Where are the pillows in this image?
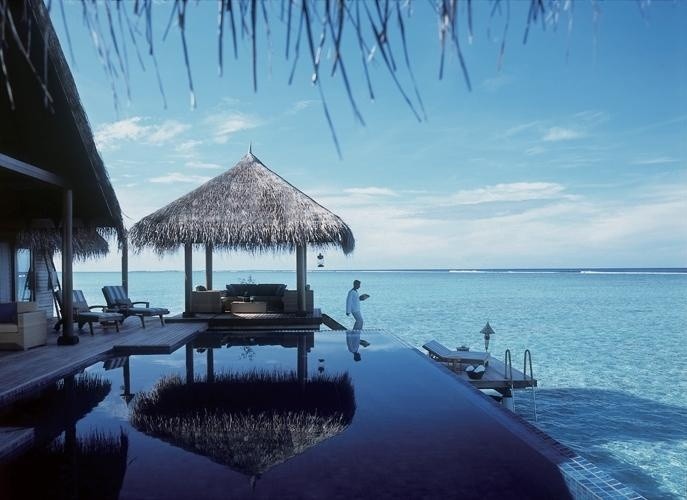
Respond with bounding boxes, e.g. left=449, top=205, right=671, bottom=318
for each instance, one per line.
left=0, top=302, right=18, bottom=324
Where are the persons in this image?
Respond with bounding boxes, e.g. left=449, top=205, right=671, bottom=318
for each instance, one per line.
left=346, top=280, right=368, bottom=330
left=346, top=330, right=371, bottom=361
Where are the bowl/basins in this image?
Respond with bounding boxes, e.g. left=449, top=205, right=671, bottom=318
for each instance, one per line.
left=466, top=371, right=484, bottom=380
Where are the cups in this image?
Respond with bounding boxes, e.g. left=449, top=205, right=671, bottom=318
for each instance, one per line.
left=250, top=296, right=253, bottom=303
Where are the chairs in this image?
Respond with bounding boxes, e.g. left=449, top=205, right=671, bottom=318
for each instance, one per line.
left=0, top=300, right=49, bottom=351
left=54, top=285, right=171, bottom=337
left=422, top=339, right=491, bottom=374
left=191, top=285, right=223, bottom=314
left=282, top=285, right=314, bottom=314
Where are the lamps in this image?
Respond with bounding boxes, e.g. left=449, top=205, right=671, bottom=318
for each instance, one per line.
left=479, top=321, right=496, bottom=352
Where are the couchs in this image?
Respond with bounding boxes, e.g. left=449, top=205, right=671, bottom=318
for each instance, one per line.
left=220, top=283, right=287, bottom=310
